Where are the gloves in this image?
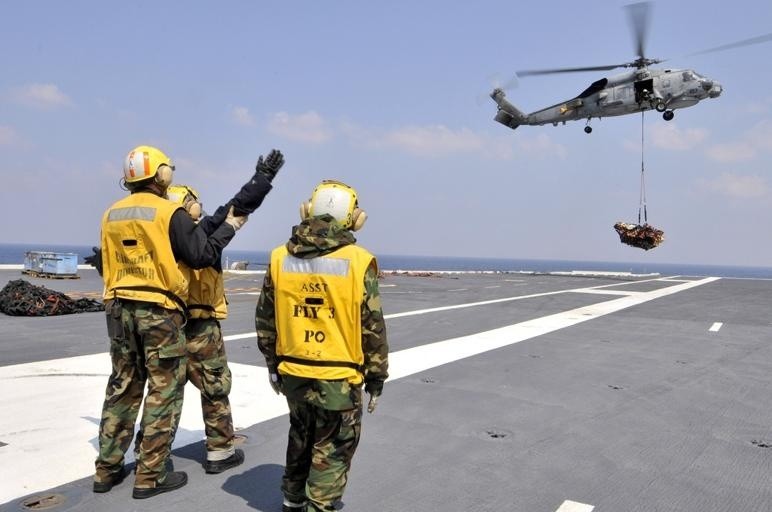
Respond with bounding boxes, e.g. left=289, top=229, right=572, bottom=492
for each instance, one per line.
left=225, top=204, right=250, bottom=233
left=83, top=245, right=101, bottom=269
left=365, top=387, right=381, bottom=413
left=267, top=372, right=287, bottom=396
left=256, top=147, right=286, bottom=184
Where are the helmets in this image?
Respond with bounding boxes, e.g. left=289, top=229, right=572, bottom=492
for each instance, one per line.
left=309, top=178, right=359, bottom=230
left=166, top=182, right=201, bottom=208
left=123, top=145, right=176, bottom=184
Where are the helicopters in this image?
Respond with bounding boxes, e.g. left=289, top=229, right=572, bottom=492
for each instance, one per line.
left=490, top=0, right=772, bottom=133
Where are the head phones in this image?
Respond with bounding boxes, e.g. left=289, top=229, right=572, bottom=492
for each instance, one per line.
left=300, top=199, right=366, bottom=232
left=123, top=165, right=173, bottom=190
left=186, top=200, right=202, bottom=221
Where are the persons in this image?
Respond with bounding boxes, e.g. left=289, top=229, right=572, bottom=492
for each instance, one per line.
left=83, top=145, right=285, bottom=473
left=93, top=144, right=247, bottom=499
left=256, top=180, right=388, bottom=511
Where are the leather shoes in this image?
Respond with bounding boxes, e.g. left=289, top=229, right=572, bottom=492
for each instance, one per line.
left=206, top=447, right=246, bottom=474
left=130, top=469, right=189, bottom=499
left=93, top=466, right=125, bottom=493
